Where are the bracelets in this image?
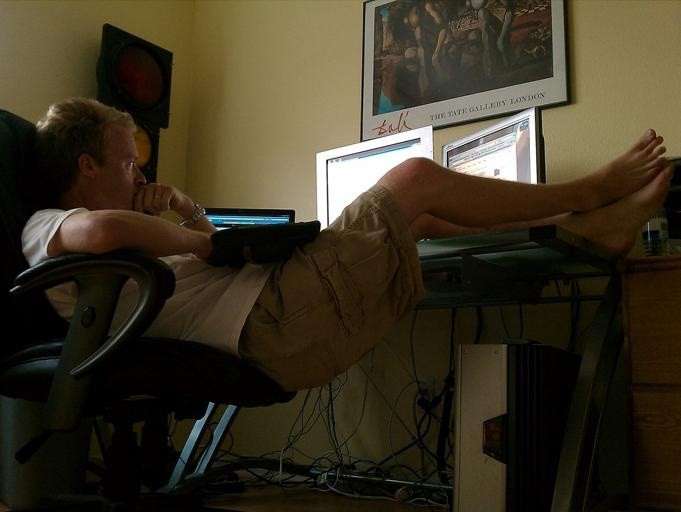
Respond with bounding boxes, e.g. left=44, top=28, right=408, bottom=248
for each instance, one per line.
left=180, top=203, right=206, bottom=227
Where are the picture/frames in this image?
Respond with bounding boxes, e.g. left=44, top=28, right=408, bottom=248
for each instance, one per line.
left=361, top=1, right=572, bottom=143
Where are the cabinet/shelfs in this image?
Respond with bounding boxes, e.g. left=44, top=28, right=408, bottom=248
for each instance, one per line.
left=606, top=246, right=679, bottom=510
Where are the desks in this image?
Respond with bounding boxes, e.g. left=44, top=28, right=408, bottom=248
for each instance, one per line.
left=161, top=221, right=630, bottom=509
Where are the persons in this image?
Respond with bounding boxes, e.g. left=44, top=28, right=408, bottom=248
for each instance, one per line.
left=16, top=92, right=679, bottom=393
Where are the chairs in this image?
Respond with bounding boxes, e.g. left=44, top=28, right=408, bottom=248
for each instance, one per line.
left=0, top=107, right=295, bottom=508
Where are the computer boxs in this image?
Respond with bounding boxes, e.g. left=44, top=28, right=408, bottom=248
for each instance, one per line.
left=452, top=340, right=580, bottom=512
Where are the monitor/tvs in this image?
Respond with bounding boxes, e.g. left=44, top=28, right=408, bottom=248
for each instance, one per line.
left=99, top=22, right=174, bottom=130
left=314, top=124, right=436, bottom=235
left=442, top=105, right=548, bottom=192
left=197, top=206, right=298, bottom=238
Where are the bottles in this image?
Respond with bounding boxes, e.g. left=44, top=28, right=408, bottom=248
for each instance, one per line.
left=638, top=212, right=670, bottom=258
